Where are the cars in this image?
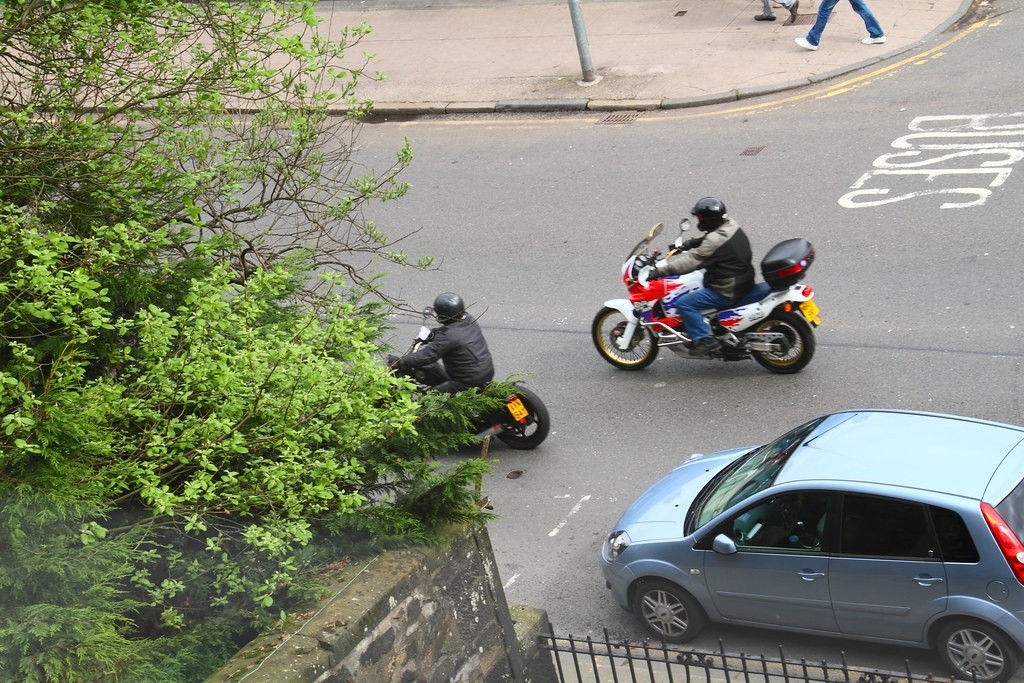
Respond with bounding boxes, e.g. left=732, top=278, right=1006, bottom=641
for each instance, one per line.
left=600, top=409, right=1024, bottom=683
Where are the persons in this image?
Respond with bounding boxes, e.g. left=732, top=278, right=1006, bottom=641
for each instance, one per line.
left=385, top=292, right=495, bottom=405
left=645, top=197, right=755, bottom=357
left=795, top=0, right=886, bottom=50
left=754, top=0, right=799, bottom=23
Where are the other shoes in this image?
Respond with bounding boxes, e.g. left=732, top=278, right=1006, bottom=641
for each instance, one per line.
left=754, top=14, right=776, bottom=21
left=789, top=0, right=799, bottom=23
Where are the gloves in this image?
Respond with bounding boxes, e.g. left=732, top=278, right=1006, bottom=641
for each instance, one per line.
left=646, top=269, right=665, bottom=281
left=387, top=355, right=400, bottom=366
left=668, top=243, right=685, bottom=255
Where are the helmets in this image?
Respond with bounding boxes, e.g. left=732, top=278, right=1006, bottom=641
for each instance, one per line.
left=691, top=197, right=727, bottom=231
left=434, top=293, right=465, bottom=325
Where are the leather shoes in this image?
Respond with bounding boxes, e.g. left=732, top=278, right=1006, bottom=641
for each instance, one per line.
left=688, top=338, right=722, bottom=357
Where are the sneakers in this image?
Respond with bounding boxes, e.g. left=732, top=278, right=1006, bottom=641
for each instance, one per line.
left=795, top=38, right=817, bottom=51
left=862, top=37, right=886, bottom=44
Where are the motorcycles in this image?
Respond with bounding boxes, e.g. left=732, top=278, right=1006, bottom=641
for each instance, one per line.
left=373, top=306, right=551, bottom=450
left=591, top=218, right=824, bottom=374
